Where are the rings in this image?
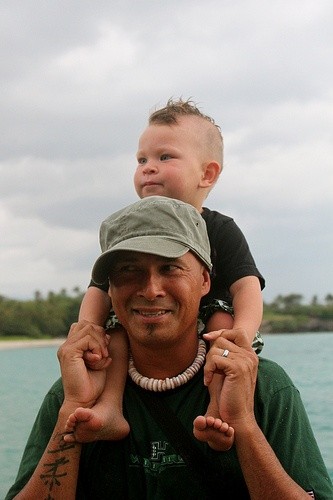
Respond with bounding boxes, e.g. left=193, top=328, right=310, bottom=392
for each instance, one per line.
left=223, top=349, right=230, bottom=358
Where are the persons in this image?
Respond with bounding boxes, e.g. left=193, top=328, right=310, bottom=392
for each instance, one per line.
left=62, top=100, right=266, bottom=453
left=5, top=195, right=333, bottom=500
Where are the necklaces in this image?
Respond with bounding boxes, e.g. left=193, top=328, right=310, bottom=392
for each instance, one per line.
left=126, top=337, right=207, bottom=392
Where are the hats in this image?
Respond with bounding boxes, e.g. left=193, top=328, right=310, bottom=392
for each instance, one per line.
left=91, top=196, right=213, bottom=284
